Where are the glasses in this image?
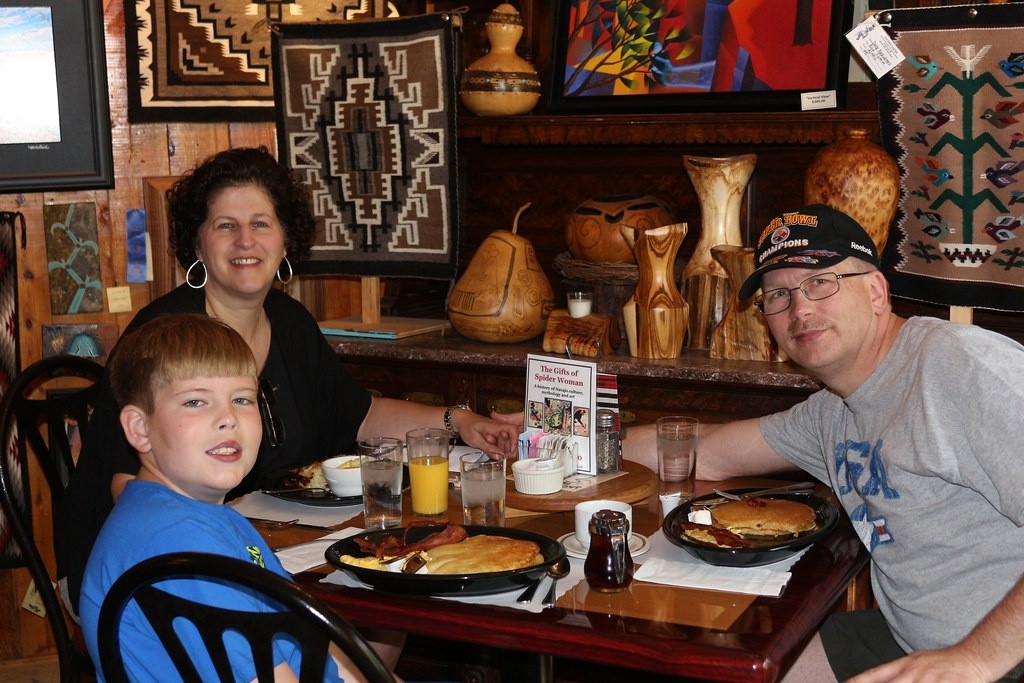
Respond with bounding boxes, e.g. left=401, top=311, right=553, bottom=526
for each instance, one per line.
left=755, top=270, right=874, bottom=316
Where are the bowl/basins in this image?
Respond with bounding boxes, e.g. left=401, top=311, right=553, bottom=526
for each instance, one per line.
left=662, top=488, right=833, bottom=567
left=321, top=455, right=376, bottom=496
left=575, top=499, right=632, bottom=551
left=511, top=458, right=565, bottom=495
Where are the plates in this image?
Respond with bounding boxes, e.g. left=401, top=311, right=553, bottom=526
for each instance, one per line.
left=324, top=523, right=567, bottom=596
left=402, top=445, right=489, bottom=472
left=260, top=465, right=410, bottom=507
left=557, top=532, right=652, bottom=559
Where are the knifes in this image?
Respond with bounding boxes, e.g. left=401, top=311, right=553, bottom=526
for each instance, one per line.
left=693, top=490, right=814, bottom=506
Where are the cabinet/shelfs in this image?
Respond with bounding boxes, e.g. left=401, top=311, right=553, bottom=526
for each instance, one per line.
left=333, top=106, right=884, bottom=399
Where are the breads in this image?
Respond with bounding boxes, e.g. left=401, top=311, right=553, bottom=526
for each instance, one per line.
left=295, top=461, right=327, bottom=492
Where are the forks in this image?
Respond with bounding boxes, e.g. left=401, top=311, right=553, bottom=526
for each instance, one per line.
left=714, top=483, right=815, bottom=502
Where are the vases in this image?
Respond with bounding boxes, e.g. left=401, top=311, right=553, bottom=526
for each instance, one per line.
left=622, top=221, right=690, bottom=358
left=680, top=153, right=759, bottom=353
left=804, top=125, right=902, bottom=255
left=709, top=244, right=788, bottom=362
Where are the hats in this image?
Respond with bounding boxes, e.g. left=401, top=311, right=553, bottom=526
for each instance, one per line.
left=737, top=202, right=879, bottom=303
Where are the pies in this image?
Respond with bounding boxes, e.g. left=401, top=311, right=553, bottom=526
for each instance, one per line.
left=417, top=534, right=544, bottom=573
left=689, top=497, right=816, bottom=535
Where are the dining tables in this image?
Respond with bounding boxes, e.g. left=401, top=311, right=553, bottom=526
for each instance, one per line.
left=252, top=472, right=870, bottom=683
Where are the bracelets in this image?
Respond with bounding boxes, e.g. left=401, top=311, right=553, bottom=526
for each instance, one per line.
left=444, top=404, right=473, bottom=437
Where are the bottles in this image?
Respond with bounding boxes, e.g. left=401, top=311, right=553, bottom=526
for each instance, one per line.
left=596, top=408, right=619, bottom=474
left=584, top=511, right=633, bottom=592
left=805, top=123, right=900, bottom=259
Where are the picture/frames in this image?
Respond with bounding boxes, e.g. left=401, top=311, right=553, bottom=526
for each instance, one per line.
left=141, top=174, right=327, bottom=324
left=0, top=0, right=116, bottom=195
left=545, top=0, right=854, bottom=112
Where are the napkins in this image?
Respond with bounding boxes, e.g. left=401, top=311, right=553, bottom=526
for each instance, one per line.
left=272, top=526, right=366, bottom=576
left=631, top=556, right=792, bottom=597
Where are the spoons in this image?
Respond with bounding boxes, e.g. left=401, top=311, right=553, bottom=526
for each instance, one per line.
left=249, top=519, right=301, bottom=531
left=542, top=558, right=570, bottom=607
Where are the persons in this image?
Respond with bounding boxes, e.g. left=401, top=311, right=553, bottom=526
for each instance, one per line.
left=484, top=202, right=1024, bottom=682
left=79, top=313, right=405, bottom=683
left=58, top=144, right=518, bottom=591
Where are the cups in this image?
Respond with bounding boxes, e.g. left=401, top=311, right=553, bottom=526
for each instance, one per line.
left=655, top=417, right=697, bottom=501
left=358, top=437, right=403, bottom=528
left=459, top=452, right=506, bottom=527
left=567, top=291, right=592, bottom=317
left=406, top=427, right=450, bottom=522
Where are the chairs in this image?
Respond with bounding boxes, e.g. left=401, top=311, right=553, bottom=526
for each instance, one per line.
left=0, top=355, right=143, bottom=683
left=95, top=551, right=395, bottom=683
